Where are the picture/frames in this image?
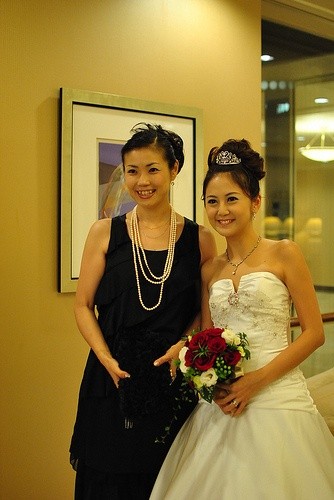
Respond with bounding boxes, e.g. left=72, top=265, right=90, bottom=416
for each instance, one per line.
left=59, top=88, right=204, bottom=294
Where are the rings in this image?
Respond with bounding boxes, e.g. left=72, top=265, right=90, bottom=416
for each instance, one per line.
left=232, top=400, right=239, bottom=408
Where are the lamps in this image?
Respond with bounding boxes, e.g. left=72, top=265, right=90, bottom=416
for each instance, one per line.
left=299, top=134, right=334, bottom=163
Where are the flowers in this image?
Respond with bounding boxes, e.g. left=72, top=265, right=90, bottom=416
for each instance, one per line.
left=177, top=323, right=252, bottom=403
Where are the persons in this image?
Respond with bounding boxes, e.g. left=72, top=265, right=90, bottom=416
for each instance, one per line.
left=68, top=123, right=220, bottom=500
left=148, top=136, right=334, bottom=500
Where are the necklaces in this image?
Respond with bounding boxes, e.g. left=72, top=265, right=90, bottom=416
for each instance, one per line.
left=224, top=234, right=262, bottom=275
left=128, top=201, right=178, bottom=310
